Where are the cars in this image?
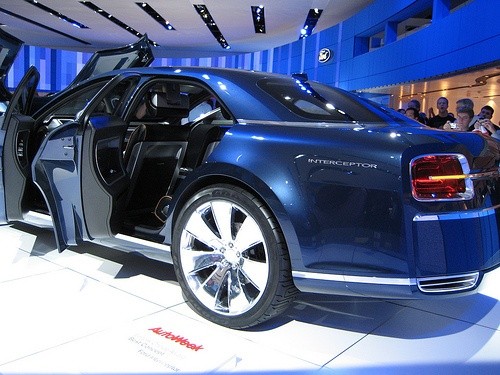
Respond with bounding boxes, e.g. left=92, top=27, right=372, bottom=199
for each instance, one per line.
left=1, top=65, right=500, bottom=331
left=0, top=27, right=155, bottom=146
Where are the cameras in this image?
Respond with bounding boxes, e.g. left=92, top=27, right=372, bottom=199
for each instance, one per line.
left=449, top=123, right=457, bottom=128
left=479, top=119, right=490, bottom=125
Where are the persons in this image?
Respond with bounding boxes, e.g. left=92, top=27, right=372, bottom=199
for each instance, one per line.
left=398, top=97, right=500, bottom=140
